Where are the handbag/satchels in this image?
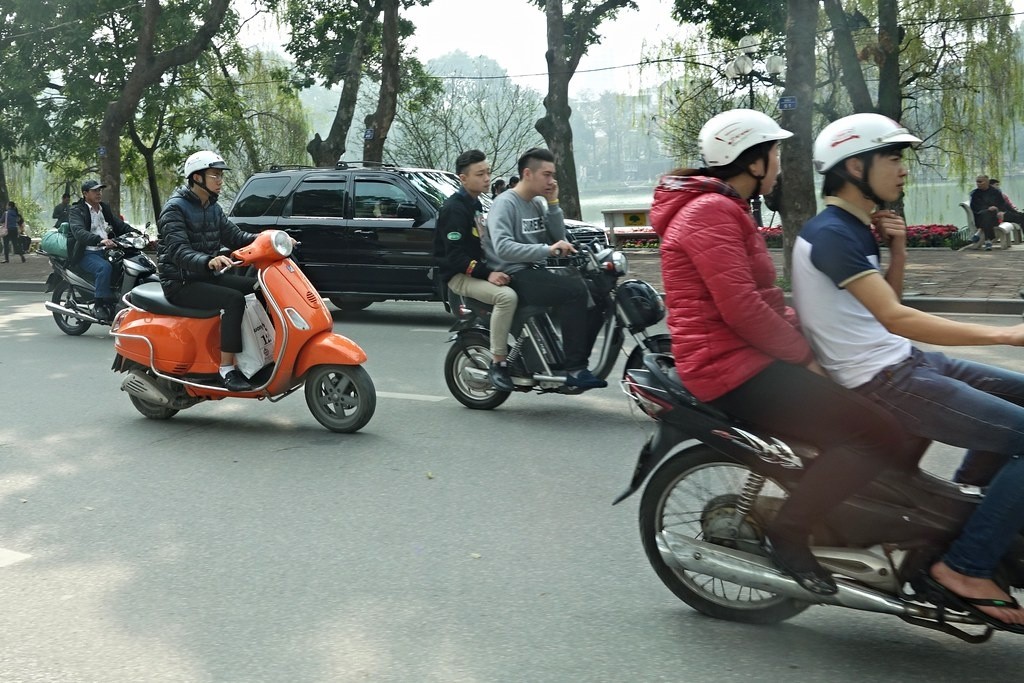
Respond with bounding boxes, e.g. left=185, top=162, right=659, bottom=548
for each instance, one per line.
left=0, top=226, right=8, bottom=237
left=221, top=293, right=276, bottom=379
left=19, top=233, right=31, bottom=250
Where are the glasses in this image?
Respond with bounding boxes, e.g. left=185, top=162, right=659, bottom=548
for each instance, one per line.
left=207, top=173, right=225, bottom=181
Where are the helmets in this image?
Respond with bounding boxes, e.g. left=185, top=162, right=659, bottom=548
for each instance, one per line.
left=813, top=112, right=923, bottom=172
left=185, top=151, right=232, bottom=178
left=697, top=108, right=794, bottom=166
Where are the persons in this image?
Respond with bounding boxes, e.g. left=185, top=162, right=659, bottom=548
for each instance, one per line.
left=433, top=146, right=609, bottom=391
left=649, top=109, right=909, bottom=595
left=157, top=150, right=297, bottom=392
left=792, top=113, right=1024, bottom=633
left=970, top=173, right=1024, bottom=250
left=0, top=180, right=149, bottom=321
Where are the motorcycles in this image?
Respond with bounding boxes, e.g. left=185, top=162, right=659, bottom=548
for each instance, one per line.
left=35, top=221, right=161, bottom=335
left=620, top=290, right=1024, bottom=645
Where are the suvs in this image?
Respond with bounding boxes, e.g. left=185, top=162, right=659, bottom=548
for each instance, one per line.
left=226, top=161, right=609, bottom=314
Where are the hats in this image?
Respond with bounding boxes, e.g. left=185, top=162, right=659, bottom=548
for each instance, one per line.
left=81, top=179, right=107, bottom=190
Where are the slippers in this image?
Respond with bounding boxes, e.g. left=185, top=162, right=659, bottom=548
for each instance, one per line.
left=927, top=562, right=1024, bottom=636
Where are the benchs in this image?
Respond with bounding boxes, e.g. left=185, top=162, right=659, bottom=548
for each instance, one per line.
left=601, top=208, right=662, bottom=247
left=1011, top=222, right=1023, bottom=245
left=25, top=225, right=43, bottom=253
left=959, top=200, right=1014, bottom=250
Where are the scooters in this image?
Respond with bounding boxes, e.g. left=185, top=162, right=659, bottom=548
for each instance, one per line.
left=108, top=228, right=377, bottom=433
left=444, top=239, right=672, bottom=410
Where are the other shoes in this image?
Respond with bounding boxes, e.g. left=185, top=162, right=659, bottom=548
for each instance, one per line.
left=21, top=256, right=25, bottom=262
left=92, top=305, right=109, bottom=319
left=985, top=242, right=992, bottom=250
left=971, top=234, right=980, bottom=243
left=2, top=259, right=10, bottom=263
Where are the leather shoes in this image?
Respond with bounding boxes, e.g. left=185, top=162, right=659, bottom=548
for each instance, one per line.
left=218, top=369, right=250, bottom=391
left=565, top=370, right=608, bottom=389
left=488, top=361, right=516, bottom=392
left=772, top=529, right=839, bottom=596
